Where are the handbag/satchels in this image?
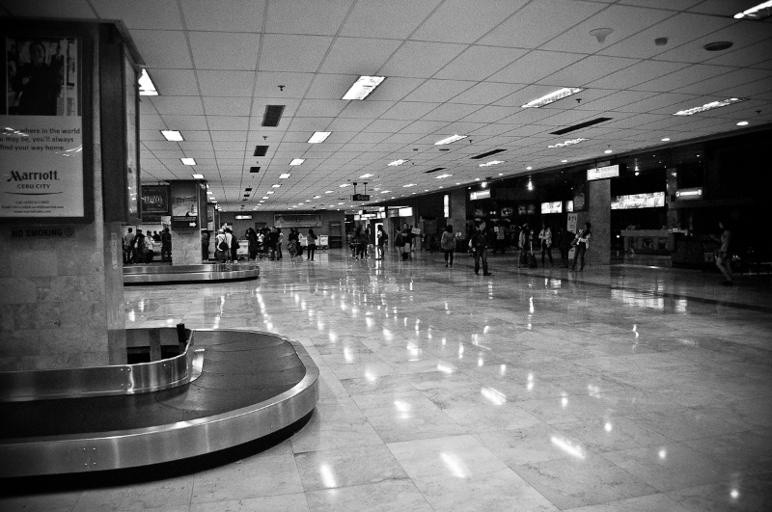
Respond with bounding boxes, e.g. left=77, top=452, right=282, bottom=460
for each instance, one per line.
left=235, top=243, right=240, bottom=250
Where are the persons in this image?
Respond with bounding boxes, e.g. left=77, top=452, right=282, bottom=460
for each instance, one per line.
left=440, top=224, right=457, bottom=268
left=202, top=230, right=210, bottom=262
left=245, top=224, right=304, bottom=261
left=307, top=229, right=317, bottom=260
left=213, top=223, right=240, bottom=264
left=346, top=221, right=422, bottom=260
left=7, top=37, right=68, bottom=116
left=516, top=221, right=592, bottom=271
left=121, top=227, right=172, bottom=264
left=470, top=219, right=492, bottom=278
left=709, top=220, right=734, bottom=287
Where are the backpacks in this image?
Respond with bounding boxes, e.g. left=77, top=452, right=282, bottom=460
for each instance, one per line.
left=218, top=237, right=228, bottom=250
